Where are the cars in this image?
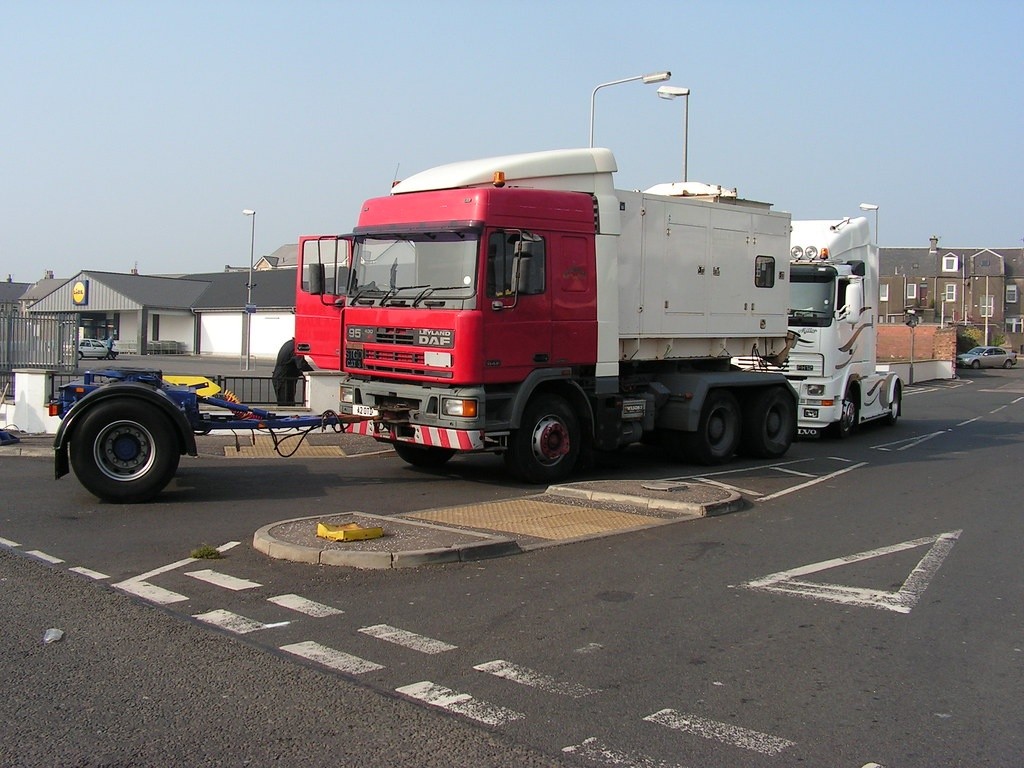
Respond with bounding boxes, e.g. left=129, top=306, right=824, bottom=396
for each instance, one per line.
left=956, top=345, right=1017, bottom=369
left=48, top=338, right=119, bottom=360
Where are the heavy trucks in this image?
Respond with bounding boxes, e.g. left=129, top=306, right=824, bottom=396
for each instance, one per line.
left=294, top=148, right=800, bottom=486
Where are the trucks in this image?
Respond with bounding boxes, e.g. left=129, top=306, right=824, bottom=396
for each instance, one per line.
left=729, top=215, right=904, bottom=440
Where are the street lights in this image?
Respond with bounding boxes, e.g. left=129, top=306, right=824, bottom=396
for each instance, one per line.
left=243, top=209, right=256, bottom=374
left=860, top=202, right=880, bottom=245
left=656, top=85, right=690, bottom=183
left=589, top=71, right=672, bottom=147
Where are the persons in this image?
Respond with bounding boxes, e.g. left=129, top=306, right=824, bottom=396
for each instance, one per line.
left=272, top=338, right=313, bottom=406
left=104, top=335, right=116, bottom=360
